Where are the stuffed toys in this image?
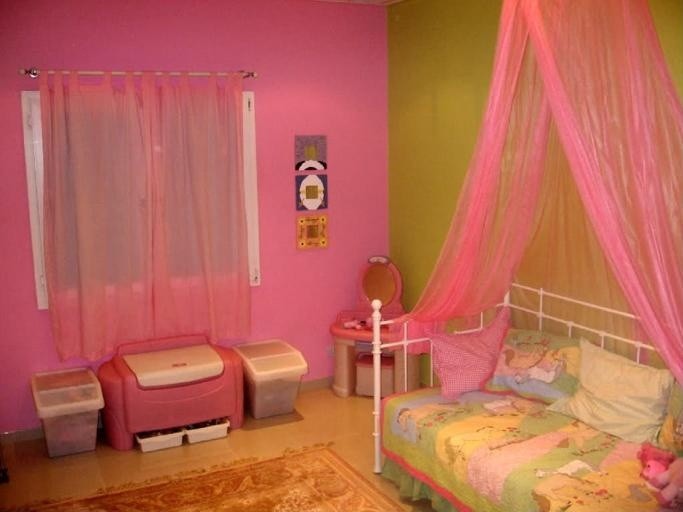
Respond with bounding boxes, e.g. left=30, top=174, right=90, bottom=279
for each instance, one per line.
left=637, top=439, right=683, bottom=509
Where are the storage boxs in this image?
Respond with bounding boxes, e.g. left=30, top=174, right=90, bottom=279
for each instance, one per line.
left=30, top=332, right=309, bottom=458
left=356, top=354, right=393, bottom=398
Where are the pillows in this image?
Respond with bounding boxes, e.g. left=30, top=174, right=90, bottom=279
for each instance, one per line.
left=424, top=305, right=674, bottom=447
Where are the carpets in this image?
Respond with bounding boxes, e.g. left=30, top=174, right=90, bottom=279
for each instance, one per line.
left=11, top=441, right=409, bottom=512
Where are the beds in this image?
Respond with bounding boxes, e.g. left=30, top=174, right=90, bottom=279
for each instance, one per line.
left=372, top=283, right=683, bottom=511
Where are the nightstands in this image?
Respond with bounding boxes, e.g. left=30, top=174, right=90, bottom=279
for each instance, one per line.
left=330, top=318, right=419, bottom=397
